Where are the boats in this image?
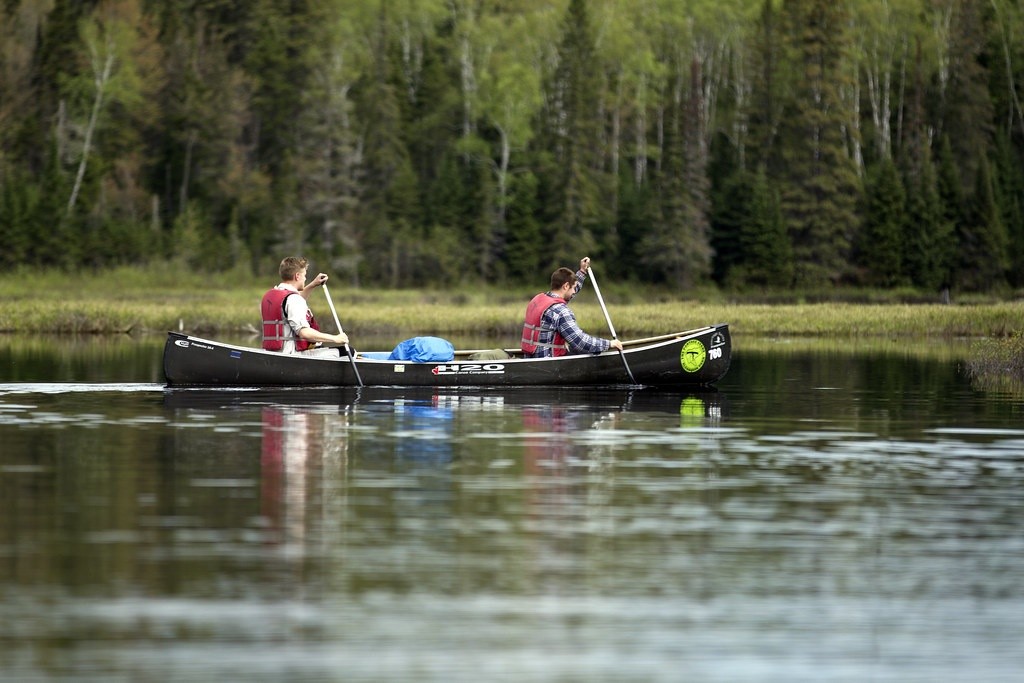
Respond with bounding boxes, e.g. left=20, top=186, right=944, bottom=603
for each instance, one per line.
left=158, top=320, right=735, bottom=389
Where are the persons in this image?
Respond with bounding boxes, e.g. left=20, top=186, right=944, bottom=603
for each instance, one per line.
left=519, top=255, right=623, bottom=358
left=261, top=256, right=359, bottom=360
